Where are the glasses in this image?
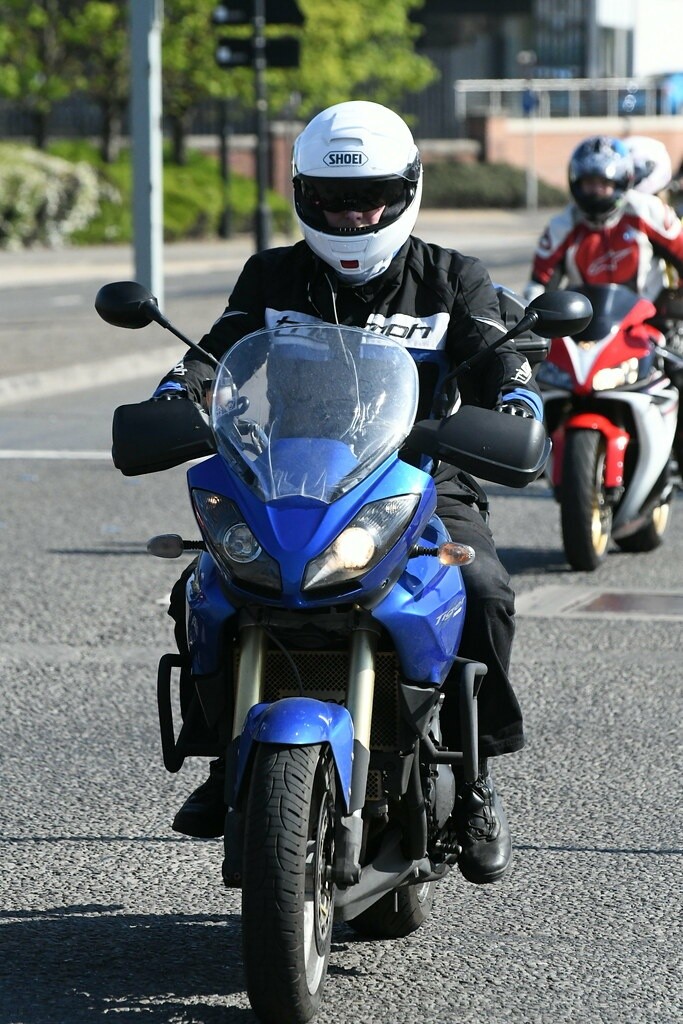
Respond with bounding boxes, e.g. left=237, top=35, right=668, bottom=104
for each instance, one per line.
left=314, top=182, right=393, bottom=214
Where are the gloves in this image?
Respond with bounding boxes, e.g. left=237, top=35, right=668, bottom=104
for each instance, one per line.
left=142, top=394, right=183, bottom=402
left=492, top=405, right=537, bottom=420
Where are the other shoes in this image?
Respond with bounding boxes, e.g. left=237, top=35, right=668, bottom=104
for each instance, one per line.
left=453, top=769, right=513, bottom=885
left=172, top=760, right=224, bottom=838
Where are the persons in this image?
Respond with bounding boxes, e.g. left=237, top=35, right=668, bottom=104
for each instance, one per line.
left=146, top=101, right=547, bottom=887
left=519, top=135, right=683, bottom=493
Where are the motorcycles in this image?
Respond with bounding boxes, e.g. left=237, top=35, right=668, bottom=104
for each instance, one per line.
left=88, top=277, right=598, bottom=1022
left=487, top=249, right=682, bottom=573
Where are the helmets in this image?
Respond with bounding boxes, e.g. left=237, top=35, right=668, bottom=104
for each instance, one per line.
left=618, top=136, right=671, bottom=196
left=290, top=101, right=422, bottom=277
left=567, top=135, right=635, bottom=219
